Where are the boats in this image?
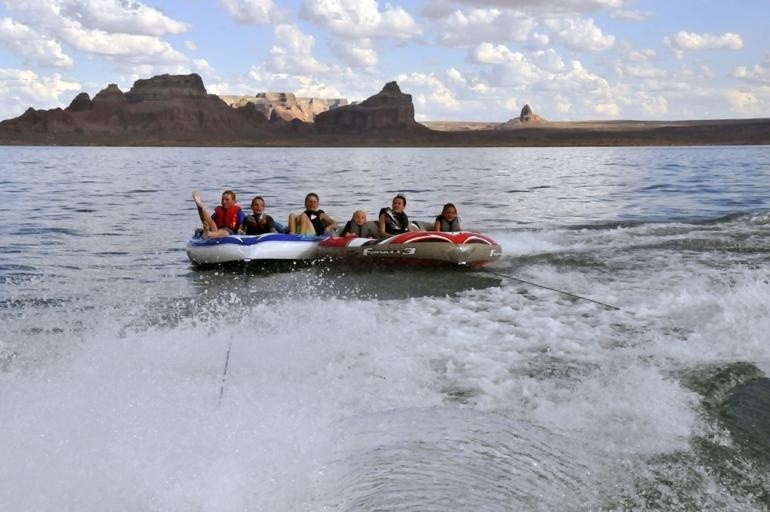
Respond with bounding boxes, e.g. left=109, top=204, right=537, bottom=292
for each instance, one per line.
left=318, top=221, right=501, bottom=267
left=185, top=221, right=329, bottom=266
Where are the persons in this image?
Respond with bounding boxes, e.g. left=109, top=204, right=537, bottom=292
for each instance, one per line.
left=379, top=194, right=409, bottom=239
left=434, top=203, right=460, bottom=232
left=240, top=197, right=280, bottom=235
left=341, top=210, right=377, bottom=240
left=192, top=190, right=246, bottom=237
left=288, top=193, right=337, bottom=237
